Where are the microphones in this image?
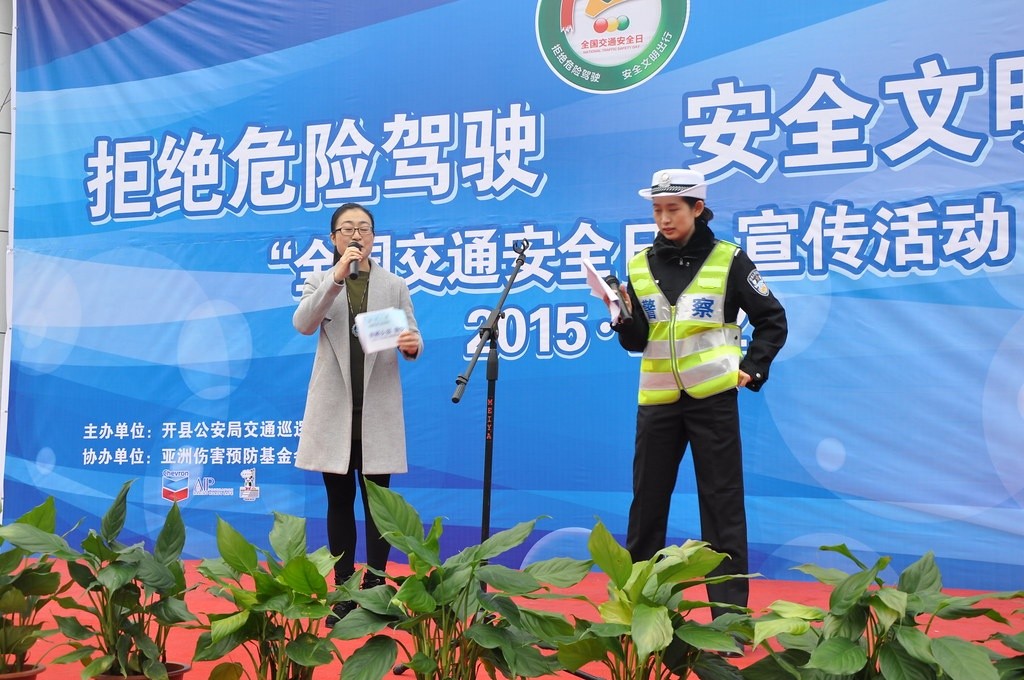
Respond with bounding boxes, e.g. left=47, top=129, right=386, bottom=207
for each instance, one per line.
left=347, top=242, right=361, bottom=279
left=605, top=275, right=633, bottom=324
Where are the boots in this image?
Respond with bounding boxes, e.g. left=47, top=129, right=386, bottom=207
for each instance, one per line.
left=360, top=573, right=385, bottom=590
left=326, top=568, right=357, bottom=627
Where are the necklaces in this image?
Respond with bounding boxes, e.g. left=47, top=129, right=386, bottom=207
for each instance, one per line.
left=346, top=279, right=369, bottom=337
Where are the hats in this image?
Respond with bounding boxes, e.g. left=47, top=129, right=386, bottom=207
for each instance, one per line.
left=639, top=169, right=706, bottom=199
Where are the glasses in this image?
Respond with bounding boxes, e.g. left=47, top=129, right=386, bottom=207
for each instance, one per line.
left=332, top=226, right=374, bottom=236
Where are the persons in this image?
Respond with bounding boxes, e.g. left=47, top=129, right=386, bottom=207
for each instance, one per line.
left=292, top=202, right=424, bottom=629
left=604, top=169, right=788, bottom=657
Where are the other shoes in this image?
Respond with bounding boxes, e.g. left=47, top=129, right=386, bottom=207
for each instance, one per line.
left=717, top=641, right=744, bottom=657
